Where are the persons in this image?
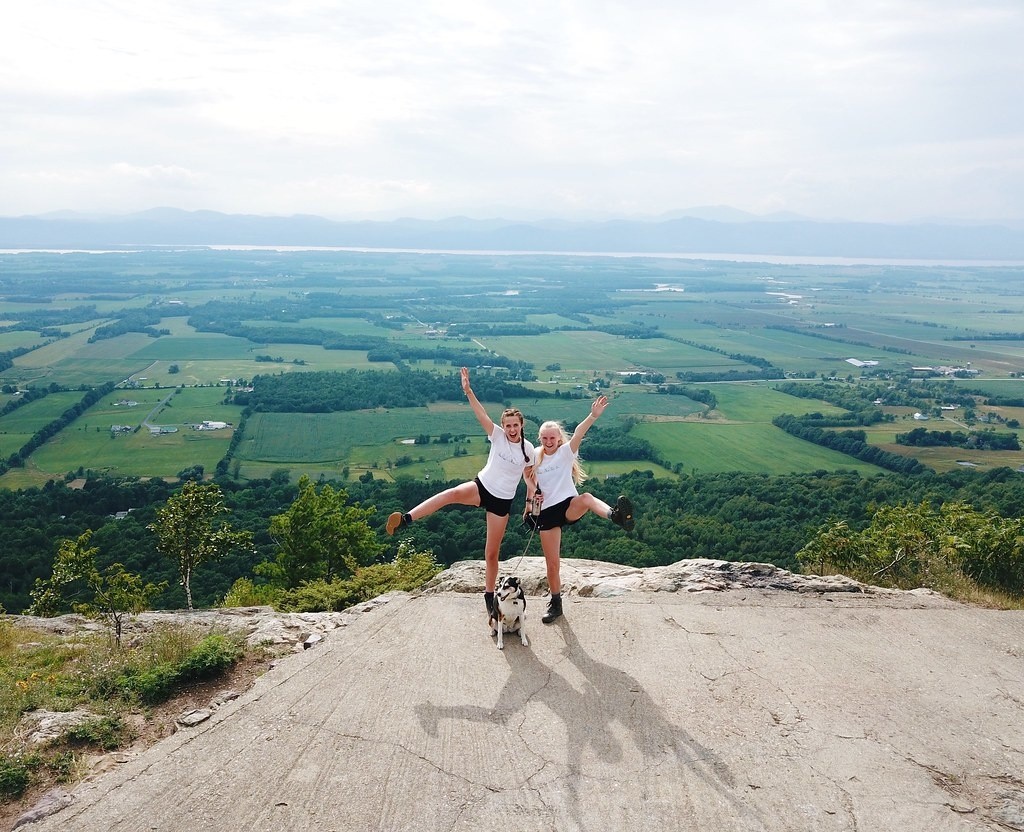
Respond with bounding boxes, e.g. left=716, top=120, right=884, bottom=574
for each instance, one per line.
left=524, top=395, right=635, bottom=624
left=387, top=368, right=544, bottom=624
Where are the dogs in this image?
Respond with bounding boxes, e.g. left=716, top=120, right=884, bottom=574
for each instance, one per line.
left=487, top=576, right=529, bottom=650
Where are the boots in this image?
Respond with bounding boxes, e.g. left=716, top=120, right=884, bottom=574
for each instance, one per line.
left=610, top=495, right=634, bottom=532
left=542, top=600, right=563, bottom=622
left=484, top=594, right=494, bottom=616
left=386, top=512, right=409, bottom=536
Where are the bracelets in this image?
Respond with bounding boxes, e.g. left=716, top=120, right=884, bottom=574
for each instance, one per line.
left=588, top=414, right=597, bottom=420
left=525, top=498, right=532, bottom=503
left=465, top=389, right=471, bottom=395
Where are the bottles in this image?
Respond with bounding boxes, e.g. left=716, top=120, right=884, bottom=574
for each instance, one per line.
left=532, top=482, right=542, bottom=515
